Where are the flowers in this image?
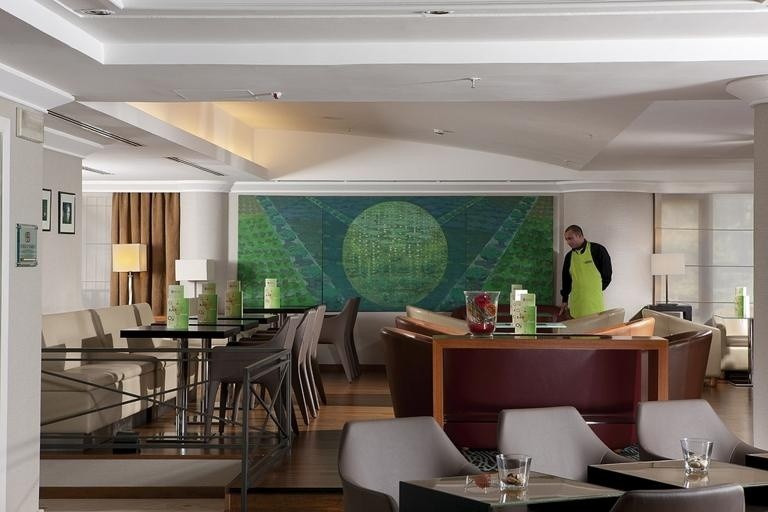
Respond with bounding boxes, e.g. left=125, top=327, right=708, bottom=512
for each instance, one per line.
left=472, top=292, right=497, bottom=318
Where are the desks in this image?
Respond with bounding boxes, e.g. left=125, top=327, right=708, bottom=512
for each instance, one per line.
left=497, top=322, right=566, bottom=328
left=587, top=456, right=768, bottom=512
left=431, top=333, right=672, bottom=429
left=398, top=469, right=630, bottom=510
left=150, top=319, right=259, bottom=425
left=117, top=325, right=240, bottom=443
left=714, top=313, right=753, bottom=374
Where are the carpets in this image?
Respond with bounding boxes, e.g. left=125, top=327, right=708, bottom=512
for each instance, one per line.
left=456, top=444, right=647, bottom=472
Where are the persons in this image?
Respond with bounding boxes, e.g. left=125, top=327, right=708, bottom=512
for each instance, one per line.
left=558, top=225, right=612, bottom=319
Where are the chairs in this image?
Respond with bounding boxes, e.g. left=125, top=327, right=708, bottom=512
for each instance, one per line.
left=380, top=305, right=470, bottom=418
left=719, top=323, right=755, bottom=374
left=635, top=396, right=767, bottom=466
left=335, top=416, right=483, bottom=511
left=608, top=482, right=748, bottom=512
left=553, top=308, right=713, bottom=401
left=496, top=404, right=637, bottom=479
left=210, top=296, right=363, bottom=447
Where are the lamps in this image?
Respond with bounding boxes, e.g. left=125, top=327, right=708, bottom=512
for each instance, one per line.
left=650, top=253, right=687, bottom=306
left=112, top=242, right=149, bottom=305
left=173, top=258, right=216, bottom=299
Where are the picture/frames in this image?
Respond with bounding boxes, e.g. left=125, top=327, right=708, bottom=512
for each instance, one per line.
left=15, top=223, right=39, bottom=268
left=42, top=188, right=53, bottom=232
left=57, top=190, right=76, bottom=235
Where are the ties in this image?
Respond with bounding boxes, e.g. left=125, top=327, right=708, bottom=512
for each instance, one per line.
left=575, top=248, right=584, bottom=255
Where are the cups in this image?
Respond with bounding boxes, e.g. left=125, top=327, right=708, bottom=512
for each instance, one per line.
left=497, top=452, right=532, bottom=491
left=499, top=492, right=527, bottom=506
left=680, top=438, right=713, bottom=480
left=464, top=475, right=499, bottom=496
left=683, top=475, right=712, bottom=490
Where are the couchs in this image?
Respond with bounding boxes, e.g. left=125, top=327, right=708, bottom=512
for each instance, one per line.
left=42, top=303, right=208, bottom=436
